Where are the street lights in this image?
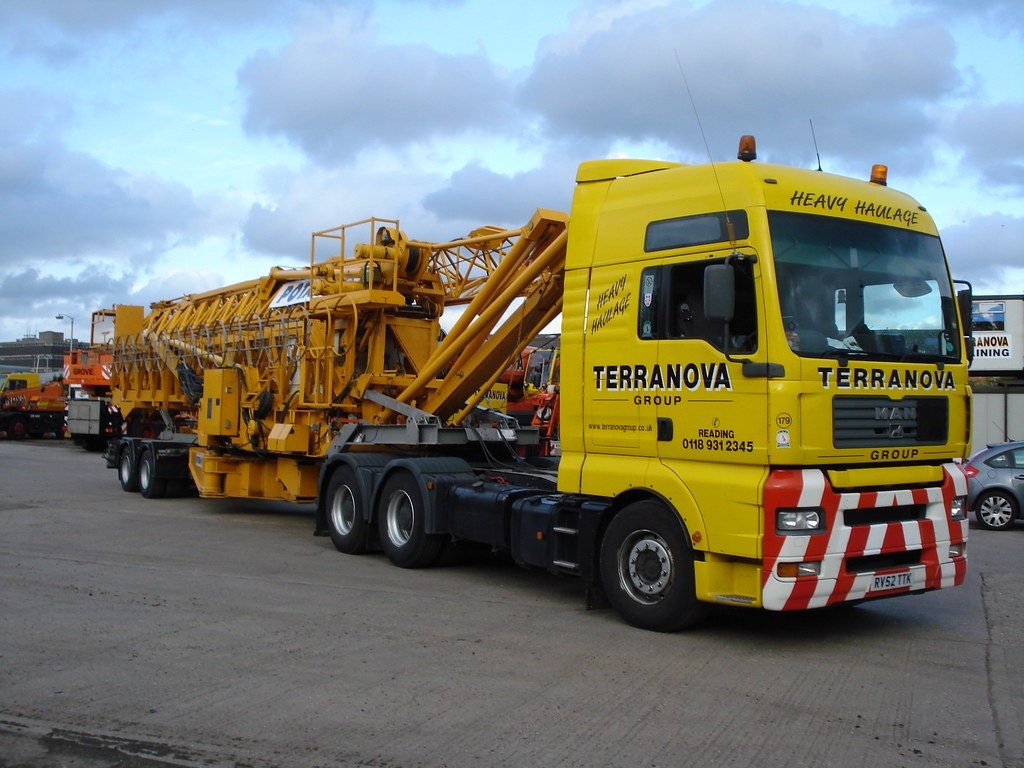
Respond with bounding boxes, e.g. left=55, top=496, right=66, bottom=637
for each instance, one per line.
left=56, top=314, right=74, bottom=355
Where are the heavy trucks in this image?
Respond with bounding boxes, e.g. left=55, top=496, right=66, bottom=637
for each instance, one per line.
left=105, top=135, right=974, bottom=633
left=0, top=372, right=68, bottom=440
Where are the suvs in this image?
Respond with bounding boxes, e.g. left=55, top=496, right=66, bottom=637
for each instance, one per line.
left=961, top=440, right=1023, bottom=531
